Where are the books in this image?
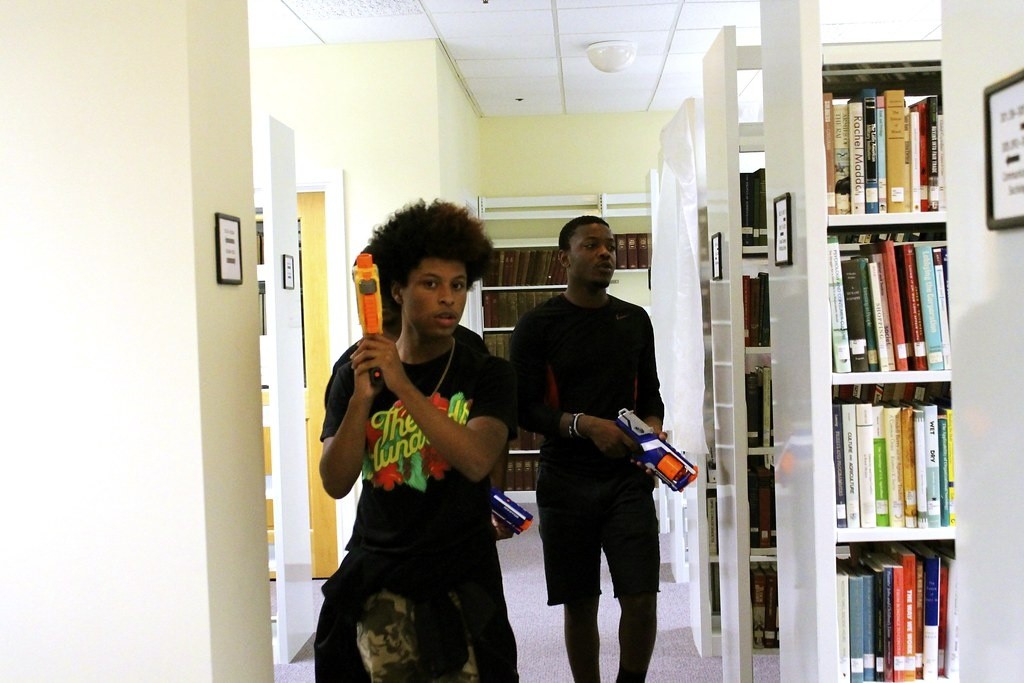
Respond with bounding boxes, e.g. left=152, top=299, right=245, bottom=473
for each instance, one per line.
left=481, top=233, right=651, bottom=491
left=740, top=167, right=779, bottom=647
left=257, top=231, right=268, bottom=337
left=821, top=87, right=964, bottom=683
left=699, top=206, right=725, bottom=617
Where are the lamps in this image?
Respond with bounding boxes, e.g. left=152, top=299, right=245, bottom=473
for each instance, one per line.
left=585, top=40, right=636, bottom=73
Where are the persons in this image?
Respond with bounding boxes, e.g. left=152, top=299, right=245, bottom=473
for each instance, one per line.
left=313, top=202, right=518, bottom=682
left=510, top=216, right=668, bottom=683
left=324, top=243, right=514, bottom=676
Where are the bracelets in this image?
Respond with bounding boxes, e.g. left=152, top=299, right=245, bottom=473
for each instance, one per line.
left=568, top=412, right=587, bottom=439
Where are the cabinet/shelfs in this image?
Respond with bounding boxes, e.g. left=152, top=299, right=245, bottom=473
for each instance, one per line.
left=469, top=1, right=1024, bottom=683
left=0, top=0, right=351, bottom=683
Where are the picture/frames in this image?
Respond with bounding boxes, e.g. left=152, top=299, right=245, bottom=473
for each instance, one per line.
left=983, top=68, right=1024, bottom=231
left=283, top=255, right=294, bottom=290
left=712, top=232, right=721, bottom=282
left=214, top=212, right=242, bottom=284
left=773, top=193, right=793, bottom=267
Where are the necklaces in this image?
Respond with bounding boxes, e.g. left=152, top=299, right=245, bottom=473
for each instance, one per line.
left=429, top=337, right=456, bottom=399
